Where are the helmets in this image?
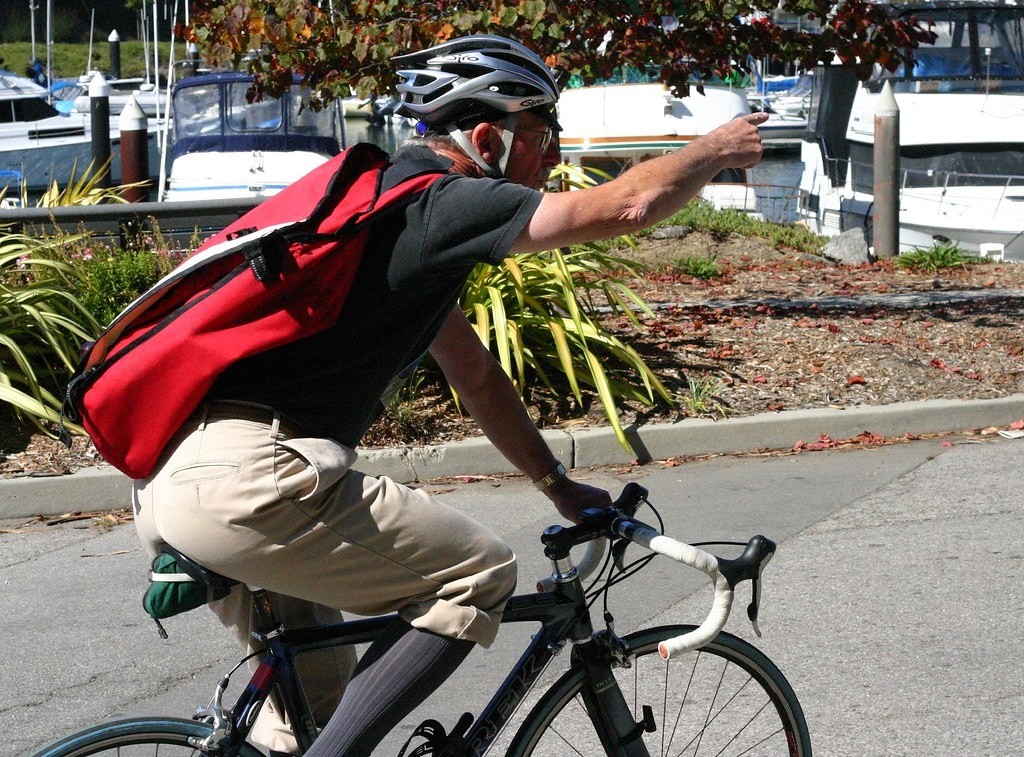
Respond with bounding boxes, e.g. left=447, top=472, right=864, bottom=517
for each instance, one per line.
left=375, top=34, right=560, bottom=123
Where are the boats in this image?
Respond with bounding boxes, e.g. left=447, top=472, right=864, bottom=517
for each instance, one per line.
left=1, top=0, right=1024, bottom=271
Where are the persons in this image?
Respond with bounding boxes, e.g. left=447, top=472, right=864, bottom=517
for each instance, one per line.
left=129, top=37, right=768, bottom=757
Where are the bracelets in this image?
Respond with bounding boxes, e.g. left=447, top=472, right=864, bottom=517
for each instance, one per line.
left=533, top=459, right=566, bottom=491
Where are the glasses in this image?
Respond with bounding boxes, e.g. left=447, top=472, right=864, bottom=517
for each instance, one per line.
left=516, top=125, right=553, bottom=156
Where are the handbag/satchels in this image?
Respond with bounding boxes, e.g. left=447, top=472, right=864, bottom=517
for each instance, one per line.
left=65, top=139, right=450, bottom=480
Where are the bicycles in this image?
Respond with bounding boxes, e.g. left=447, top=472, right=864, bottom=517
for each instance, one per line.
left=29, top=481, right=815, bottom=757
